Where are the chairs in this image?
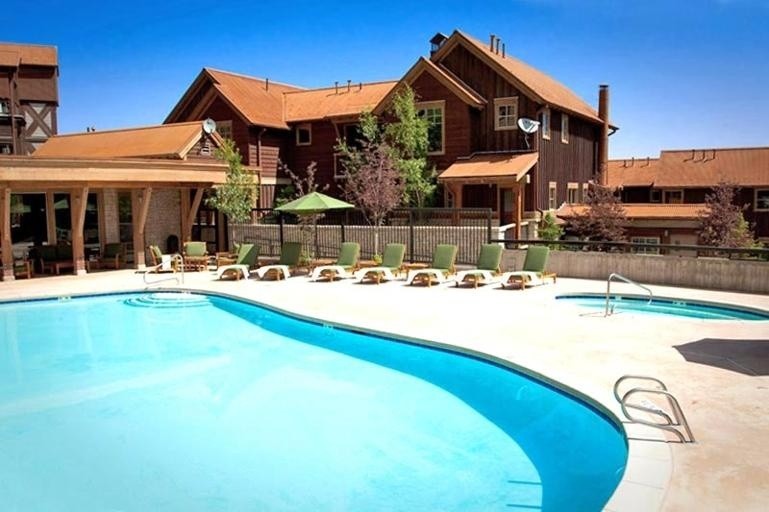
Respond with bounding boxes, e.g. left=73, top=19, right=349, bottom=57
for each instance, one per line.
left=149, top=244, right=184, bottom=277
left=316, top=243, right=362, bottom=282
left=501, top=246, right=557, bottom=290
left=258, top=244, right=302, bottom=280
left=409, top=244, right=457, bottom=287
left=218, top=244, right=261, bottom=282
left=461, top=245, right=504, bottom=289
left=182, top=241, right=208, bottom=274
left=0, top=240, right=127, bottom=280
left=358, top=244, right=406, bottom=283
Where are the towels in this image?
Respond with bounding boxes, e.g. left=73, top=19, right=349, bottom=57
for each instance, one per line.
left=161, top=254, right=171, bottom=271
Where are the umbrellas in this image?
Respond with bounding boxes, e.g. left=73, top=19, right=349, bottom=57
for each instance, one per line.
left=274, top=191, right=355, bottom=261
